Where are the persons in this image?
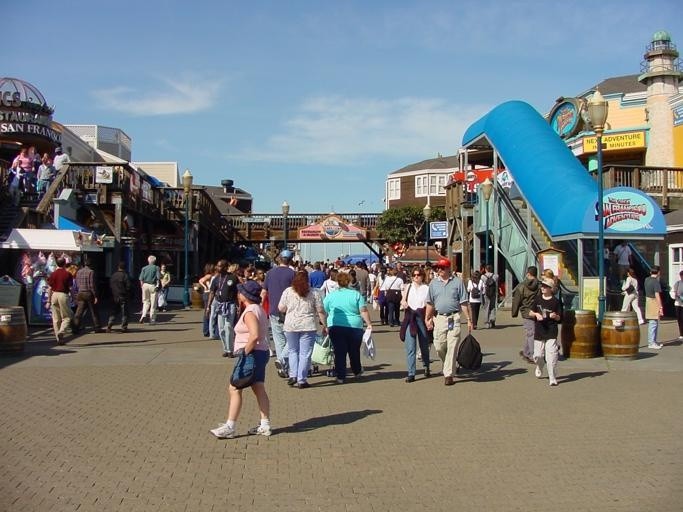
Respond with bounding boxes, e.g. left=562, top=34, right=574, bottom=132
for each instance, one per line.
left=424, top=257, right=472, bottom=386
left=404, top=257, right=502, bottom=333
left=156, top=262, right=171, bottom=312
left=604, top=239, right=683, bottom=350
left=405, top=269, right=429, bottom=383
left=1, top=146, right=131, bottom=346
left=511, top=266, right=564, bottom=386
left=199, top=249, right=404, bottom=389
left=209, top=280, right=273, bottom=439
left=138, top=255, right=164, bottom=323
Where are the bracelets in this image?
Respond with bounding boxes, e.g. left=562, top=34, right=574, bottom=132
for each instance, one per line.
left=466, top=317, right=472, bottom=320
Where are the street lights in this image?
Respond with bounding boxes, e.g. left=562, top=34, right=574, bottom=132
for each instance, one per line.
left=281, top=201, right=289, bottom=250
left=181, top=170, right=192, bottom=308
left=586, top=90, right=609, bottom=326
left=481, top=178, right=493, bottom=270
left=422, top=205, right=432, bottom=261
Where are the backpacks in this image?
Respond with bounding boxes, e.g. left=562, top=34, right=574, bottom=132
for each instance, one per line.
left=483, top=273, right=497, bottom=299
left=470, top=279, right=480, bottom=298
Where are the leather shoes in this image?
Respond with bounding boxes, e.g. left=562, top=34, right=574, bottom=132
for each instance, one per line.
left=445, top=377, right=454, bottom=385
left=287, top=377, right=297, bottom=385
left=405, top=376, right=415, bottom=382
left=424, top=368, right=429, bottom=376
left=298, top=382, right=311, bottom=389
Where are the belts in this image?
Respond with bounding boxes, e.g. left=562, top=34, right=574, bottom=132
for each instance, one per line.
left=435, top=310, right=459, bottom=317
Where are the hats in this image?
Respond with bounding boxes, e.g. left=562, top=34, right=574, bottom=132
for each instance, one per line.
left=435, top=259, right=451, bottom=267
left=279, top=250, right=293, bottom=258
left=542, top=277, right=555, bottom=288
left=236, top=280, right=262, bottom=304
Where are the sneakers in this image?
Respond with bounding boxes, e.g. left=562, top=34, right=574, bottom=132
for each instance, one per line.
left=248, top=425, right=273, bottom=436
left=638, top=319, right=683, bottom=349
left=550, top=376, right=558, bottom=386
left=535, top=366, right=543, bottom=378
left=58, top=317, right=156, bottom=345
left=336, top=377, right=343, bottom=384
left=519, top=350, right=535, bottom=364
left=222, top=352, right=235, bottom=358
left=275, top=358, right=289, bottom=378
left=209, top=423, right=235, bottom=439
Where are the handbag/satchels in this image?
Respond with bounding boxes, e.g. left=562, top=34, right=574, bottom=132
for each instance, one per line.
left=311, top=334, right=332, bottom=366
left=230, top=348, right=257, bottom=389
left=626, top=285, right=634, bottom=295
left=457, top=334, right=482, bottom=371
left=372, top=298, right=381, bottom=311
left=158, top=294, right=168, bottom=307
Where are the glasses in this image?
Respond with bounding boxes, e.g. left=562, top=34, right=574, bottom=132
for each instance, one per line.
left=412, top=274, right=421, bottom=277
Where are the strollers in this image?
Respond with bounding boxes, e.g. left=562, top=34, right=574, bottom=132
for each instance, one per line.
left=307, top=335, right=337, bottom=377
